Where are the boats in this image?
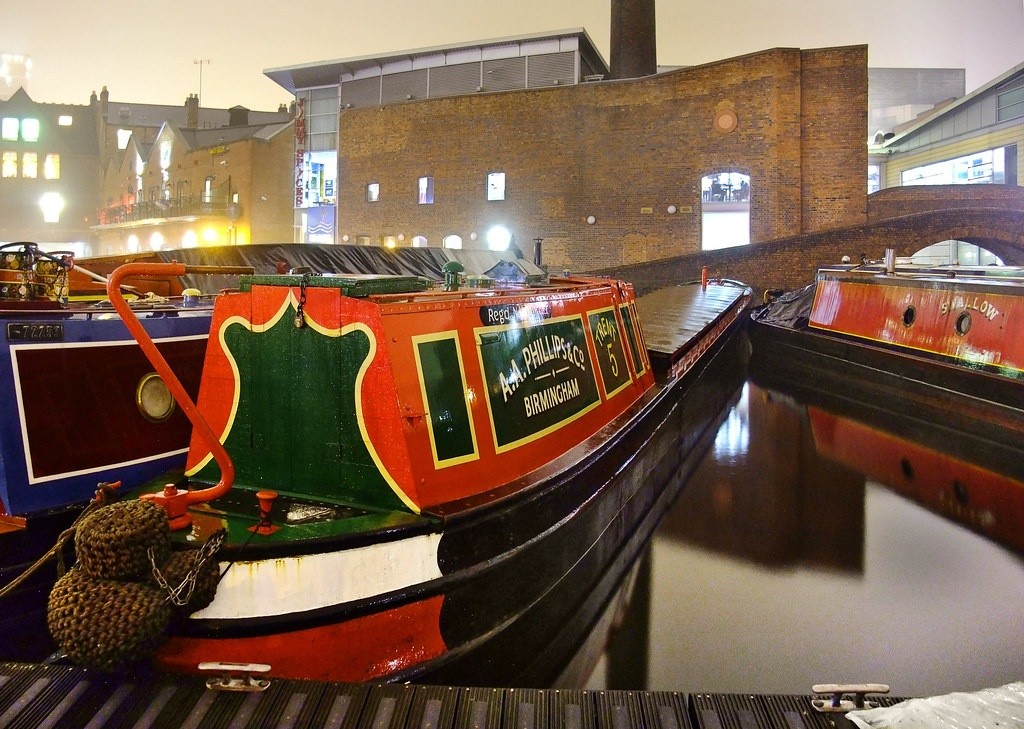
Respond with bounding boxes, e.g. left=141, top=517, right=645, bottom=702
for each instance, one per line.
left=1, top=230, right=1024, bottom=688
